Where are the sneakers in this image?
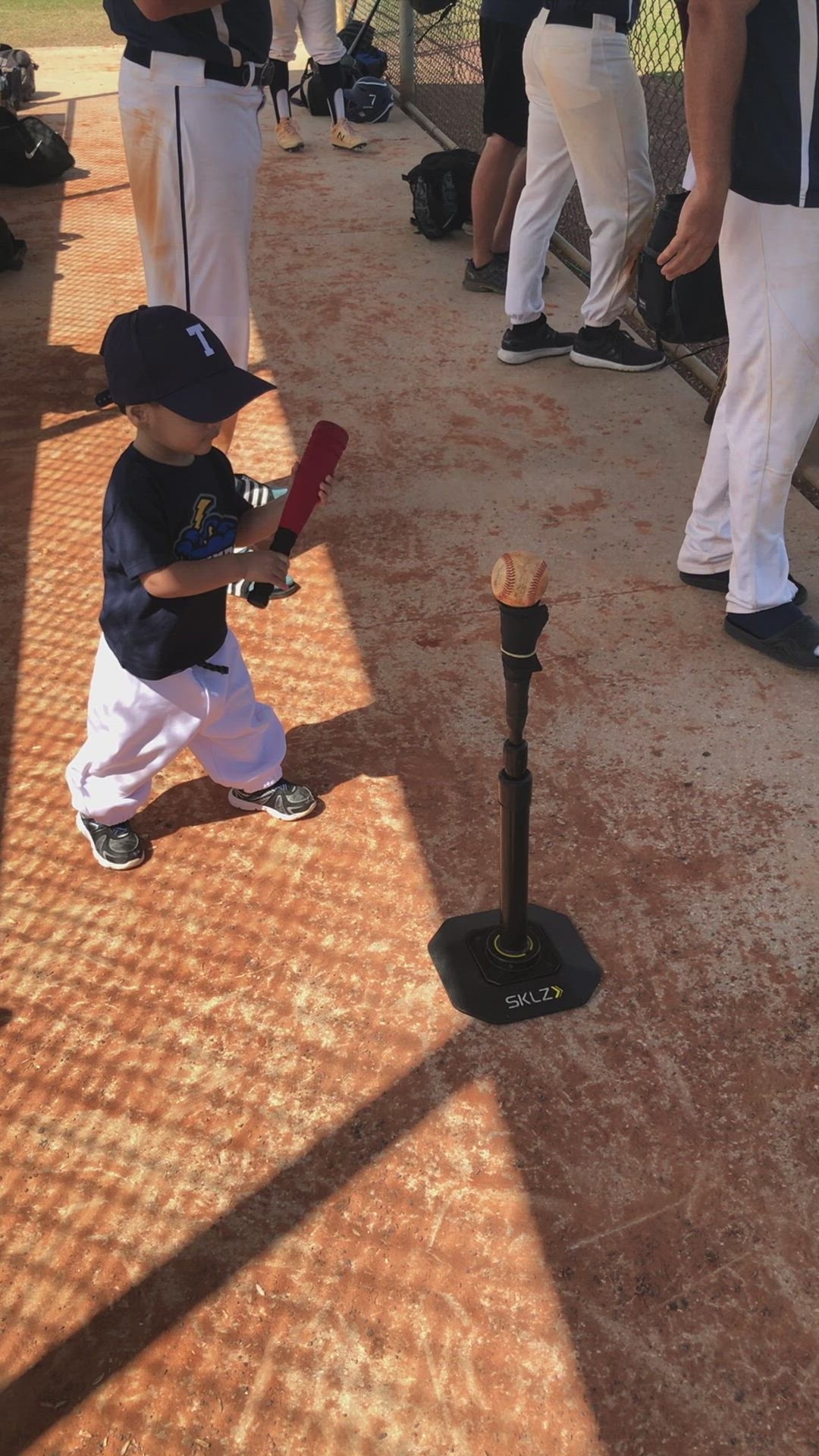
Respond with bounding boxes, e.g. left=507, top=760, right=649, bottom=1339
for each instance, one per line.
left=275, top=117, right=305, bottom=152
left=491, top=251, right=550, bottom=280
left=497, top=312, right=578, bottom=364
left=461, top=256, right=508, bottom=295
left=569, top=319, right=666, bottom=372
left=228, top=777, right=317, bottom=821
left=330, top=118, right=367, bottom=152
left=75, top=811, right=145, bottom=871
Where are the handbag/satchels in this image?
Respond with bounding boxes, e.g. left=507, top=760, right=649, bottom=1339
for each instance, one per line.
left=402, top=149, right=480, bottom=241
left=0, top=42, right=76, bottom=187
left=288, top=20, right=394, bottom=124
left=0, top=216, right=27, bottom=271
left=637, top=191, right=728, bottom=352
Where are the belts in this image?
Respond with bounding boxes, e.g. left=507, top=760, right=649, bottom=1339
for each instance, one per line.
left=124, top=43, right=275, bottom=86
left=546, top=11, right=629, bottom=35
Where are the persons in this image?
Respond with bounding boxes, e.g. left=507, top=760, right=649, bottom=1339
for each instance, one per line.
left=266, top=0, right=368, bottom=152
left=461, top=0, right=549, bottom=296
left=101, top=0, right=299, bottom=600
left=497, top=0, right=666, bottom=372
left=64, top=304, right=336, bottom=869
left=655, top=0, right=819, bottom=672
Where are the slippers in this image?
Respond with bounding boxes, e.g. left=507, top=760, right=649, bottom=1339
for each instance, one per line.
left=723, top=613, right=818, bottom=671
left=679, top=569, right=808, bottom=608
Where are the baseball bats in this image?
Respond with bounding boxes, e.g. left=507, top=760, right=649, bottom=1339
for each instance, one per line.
left=346, top=0, right=357, bottom=22
left=345, top=0, right=381, bottom=55
left=246, top=419, right=349, bottom=610
left=703, top=358, right=729, bottom=426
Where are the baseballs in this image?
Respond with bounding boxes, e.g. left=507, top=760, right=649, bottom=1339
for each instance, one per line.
left=491, top=551, right=550, bottom=607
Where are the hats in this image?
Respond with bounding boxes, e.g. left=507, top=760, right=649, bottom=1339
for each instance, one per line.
left=95, top=304, right=278, bottom=423
